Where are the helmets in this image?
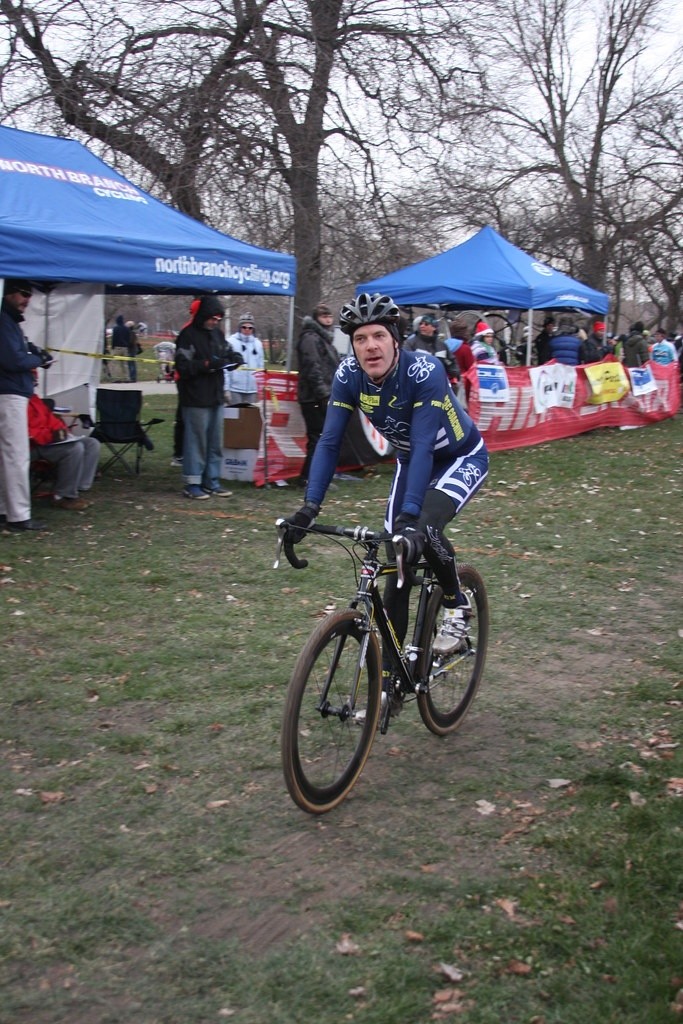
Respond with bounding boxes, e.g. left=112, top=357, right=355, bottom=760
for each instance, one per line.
left=339, top=292, right=401, bottom=332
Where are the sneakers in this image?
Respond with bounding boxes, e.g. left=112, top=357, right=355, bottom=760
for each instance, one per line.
left=183, top=484, right=210, bottom=500
left=354, top=691, right=405, bottom=726
left=171, top=456, right=185, bottom=467
left=431, top=590, right=472, bottom=655
left=205, top=486, right=233, bottom=496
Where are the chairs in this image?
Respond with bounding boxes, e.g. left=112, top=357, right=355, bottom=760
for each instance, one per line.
left=26, top=436, right=59, bottom=498
left=77, top=388, right=165, bottom=476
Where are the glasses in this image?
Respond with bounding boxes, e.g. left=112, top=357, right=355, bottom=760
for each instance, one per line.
left=419, top=322, right=429, bottom=326
left=240, top=326, right=254, bottom=329
left=210, top=315, right=223, bottom=323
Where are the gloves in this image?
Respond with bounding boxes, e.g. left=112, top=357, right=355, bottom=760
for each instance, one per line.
left=393, top=515, right=427, bottom=557
left=280, top=508, right=314, bottom=543
left=53, top=428, right=67, bottom=443
left=39, top=350, right=53, bottom=368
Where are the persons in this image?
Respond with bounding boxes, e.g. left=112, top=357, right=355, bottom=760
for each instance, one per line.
left=295, top=303, right=376, bottom=489
left=280, top=292, right=493, bottom=723
left=173, top=296, right=243, bottom=499
left=404, top=311, right=683, bottom=436
left=0, top=279, right=52, bottom=531
left=170, top=298, right=266, bottom=466
left=103, top=314, right=139, bottom=383
left=28, top=367, right=101, bottom=511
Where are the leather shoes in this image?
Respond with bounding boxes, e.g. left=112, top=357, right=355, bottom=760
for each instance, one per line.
left=53, top=494, right=94, bottom=511
left=9, top=518, right=47, bottom=532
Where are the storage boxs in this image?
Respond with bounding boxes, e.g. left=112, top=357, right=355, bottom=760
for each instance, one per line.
left=222, top=402, right=266, bottom=449
left=219, top=447, right=260, bottom=481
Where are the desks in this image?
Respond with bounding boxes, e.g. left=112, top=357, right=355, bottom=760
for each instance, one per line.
left=52, top=411, right=81, bottom=433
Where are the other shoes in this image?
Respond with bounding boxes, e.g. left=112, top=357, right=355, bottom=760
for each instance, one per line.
left=296, top=478, right=337, bottom=491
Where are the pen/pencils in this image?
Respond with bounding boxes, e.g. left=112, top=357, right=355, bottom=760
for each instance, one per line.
left=211, top=354, right=220, bottom=361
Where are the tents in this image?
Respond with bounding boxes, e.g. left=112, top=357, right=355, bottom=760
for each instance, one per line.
left=356, top=220, right=610, bottom=367
left=0, top=125, right=296, bottom=370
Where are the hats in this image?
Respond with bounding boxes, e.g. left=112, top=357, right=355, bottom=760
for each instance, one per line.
left=421, top=313, right=438, bottom=326
left=542, top=317, right=555, bottom=327
left=449, top=317, right=469, bottom=338
left=474, top=322, right=495, bottom=336
left=592, top=321, right=607, bottom=333
left=313, top=303, right=334, bottom=317
left=238, top=312, right=256, bottom=326
left=553, top=316, right=578, bottom=336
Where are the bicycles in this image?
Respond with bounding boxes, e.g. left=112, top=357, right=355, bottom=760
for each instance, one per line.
left=274, top=518, right=490, bottom=815
